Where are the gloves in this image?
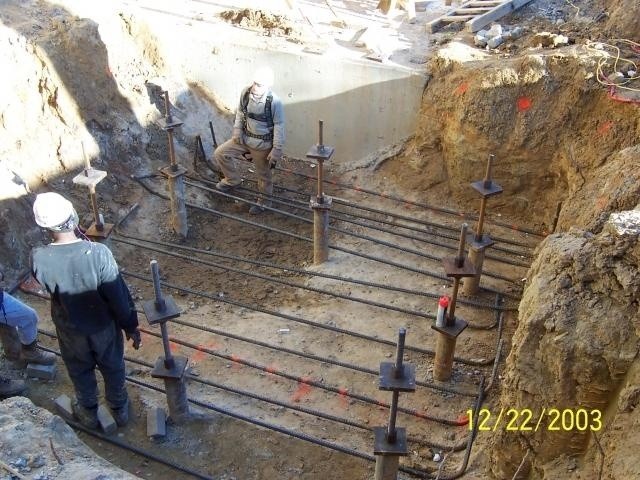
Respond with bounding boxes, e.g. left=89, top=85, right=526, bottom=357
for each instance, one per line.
left=267, top=147, right=282, bottom=167
left=232, top=128, right=244, bottom=145
left=125, top=328, right=142, bottom=351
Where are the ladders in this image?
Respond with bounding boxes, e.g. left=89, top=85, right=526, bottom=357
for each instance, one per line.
left=424, top=0, right=531, bottom=33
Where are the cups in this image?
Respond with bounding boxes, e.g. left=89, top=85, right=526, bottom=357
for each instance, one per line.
left=95, top=213, right=105, bottom=226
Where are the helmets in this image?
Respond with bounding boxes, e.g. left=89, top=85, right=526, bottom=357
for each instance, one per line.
left=33, top=191, right=80, bottom=235
left=251, top=63, right=275, bottom=87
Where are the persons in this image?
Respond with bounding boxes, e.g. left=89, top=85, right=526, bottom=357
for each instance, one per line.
left=212, top=67, right=287, bottom=215
left=0, top=290, right=57, bottom=366
left=30, top=191, right=142, bottom=430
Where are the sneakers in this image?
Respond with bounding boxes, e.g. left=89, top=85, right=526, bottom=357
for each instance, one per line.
left=248, top=198, right=274, bottom=215
left=20, top=341, right=58, bottom=366
left=108, top=397, right=131, bottom=428
left=215, top=178, right=242, bottom=192
left=71, top=395, right=99, bottom=429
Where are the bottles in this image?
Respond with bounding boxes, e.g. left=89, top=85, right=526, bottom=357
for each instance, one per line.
left=436, top=298, right=449, bottom=328
